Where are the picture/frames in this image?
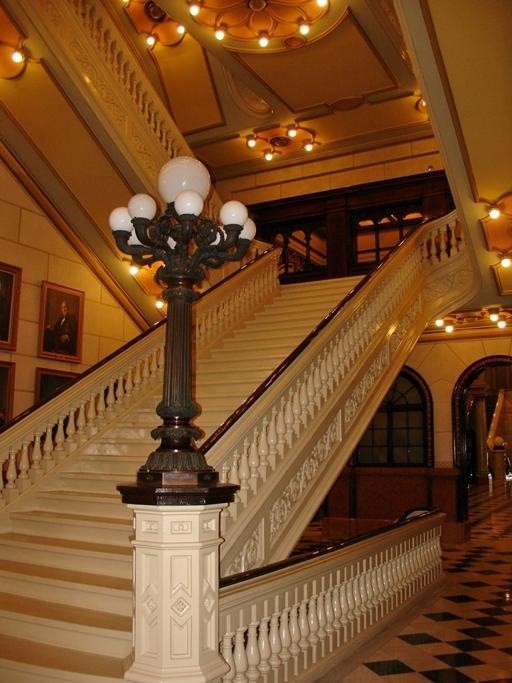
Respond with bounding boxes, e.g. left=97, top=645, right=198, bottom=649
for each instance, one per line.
left=34, top=366, right=83, bottom=406
left=1, top=260, right=24, bottom=352
left=38, top=280, right=85, bottom=364
left=0, top=360, right=16, bottom=427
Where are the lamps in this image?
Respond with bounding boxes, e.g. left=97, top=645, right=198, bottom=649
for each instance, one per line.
left=246, top=121, right=316, bottom=163
left=189, top=0, right=326, bottom=48
left=434, top=301, right=512, bottom=334
left=147, top=23, right=186, bottom=47
left=480, top=189, right=512, bottom=268
left=13, top=48, right=27, bottom=63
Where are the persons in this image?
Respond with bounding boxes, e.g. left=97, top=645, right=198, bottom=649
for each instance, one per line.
left=46, top=300, right=76, bottom=354
